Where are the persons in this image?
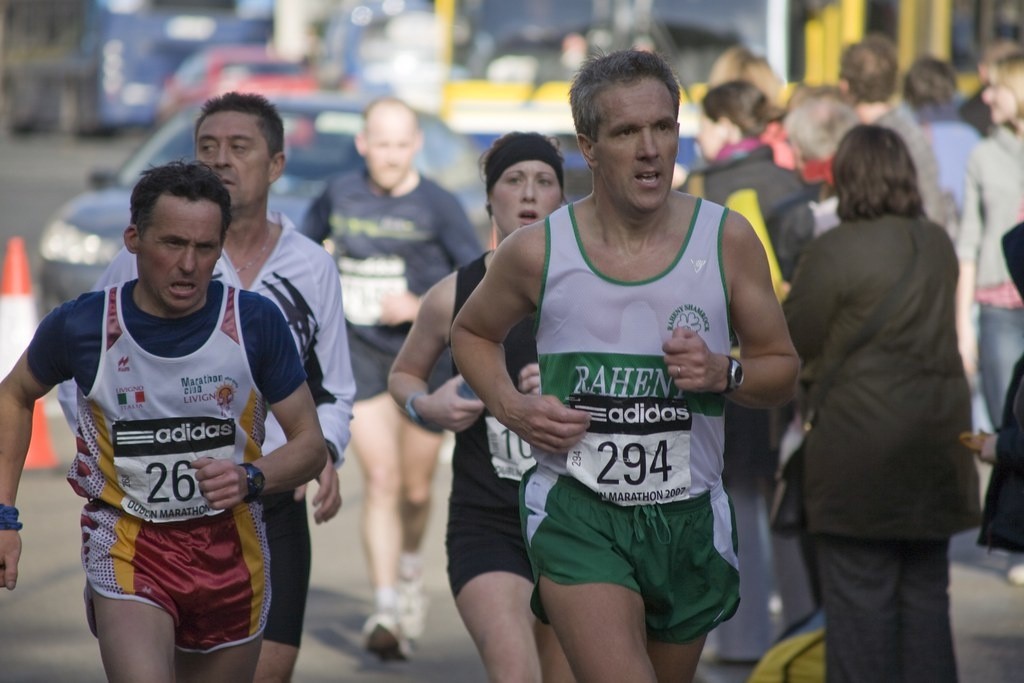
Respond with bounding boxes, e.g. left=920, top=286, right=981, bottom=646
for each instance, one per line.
left=673, top=7, right=1024, bottom=683
left=0, top=159, right=325, bottom=682
left=58, top=91, right=357, bottom=682
left=388, top=131, right=577, bottom=683
left=448, top=49, right=800, bottom=683
left=303, top=99, right=482, bottom=659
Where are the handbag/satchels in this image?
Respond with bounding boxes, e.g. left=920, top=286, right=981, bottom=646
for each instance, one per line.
left=686, top=173, right=786, bottom=363
left=768, top=422, right=809, bottom=539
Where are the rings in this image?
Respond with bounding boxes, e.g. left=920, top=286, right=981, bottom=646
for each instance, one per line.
left=677, top=366, right=681, bottom=377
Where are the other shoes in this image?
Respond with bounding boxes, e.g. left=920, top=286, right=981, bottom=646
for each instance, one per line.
left=398, top=567, right=427, bottom=639
left=362, top=616, right=412, bottom=665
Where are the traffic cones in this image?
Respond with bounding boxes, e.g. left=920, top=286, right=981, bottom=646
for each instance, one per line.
left=1, top=236, right=72, bottom=483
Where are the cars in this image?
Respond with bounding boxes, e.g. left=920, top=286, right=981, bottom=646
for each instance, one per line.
left=31, top=92, right=493, bottom=318
left=0, top=0, right=701, bottom=201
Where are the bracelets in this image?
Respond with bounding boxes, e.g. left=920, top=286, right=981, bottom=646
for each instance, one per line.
left=326, top=441, right=340, bottom=463
left=0, top=500, right=28, bottom=535
left=406, top=389, right=429, bottom=427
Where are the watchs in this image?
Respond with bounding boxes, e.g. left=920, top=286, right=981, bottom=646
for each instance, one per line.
left=238, top=461, right=267, bottom=503
left=726, top=354, right=745, bottom=396
